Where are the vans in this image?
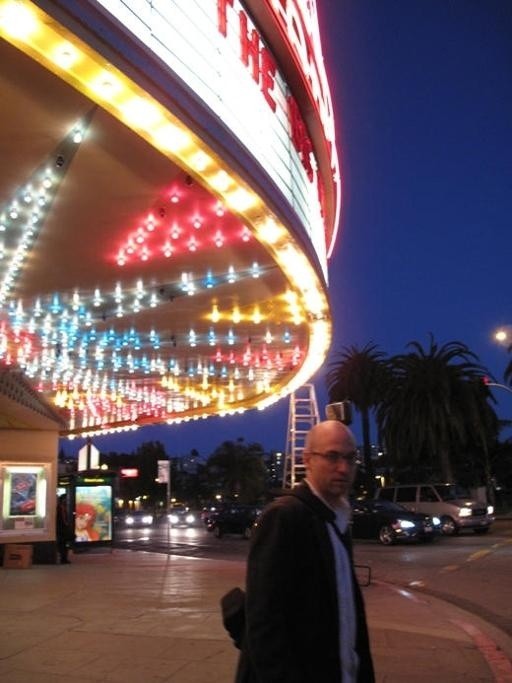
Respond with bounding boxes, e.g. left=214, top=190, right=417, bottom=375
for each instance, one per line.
left=374, top=483, right=497, bottom=536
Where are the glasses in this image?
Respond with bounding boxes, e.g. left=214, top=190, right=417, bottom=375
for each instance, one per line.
left=309, top=452, right=358, bottom=466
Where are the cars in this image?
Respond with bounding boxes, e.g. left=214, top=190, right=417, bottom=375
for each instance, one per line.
left=347, top=499, right=442, bottom=545
left=125, top=497, right=263, bottom=540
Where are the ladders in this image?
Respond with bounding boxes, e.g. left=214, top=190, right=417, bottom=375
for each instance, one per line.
left=282, top=384, right=321, bottom=490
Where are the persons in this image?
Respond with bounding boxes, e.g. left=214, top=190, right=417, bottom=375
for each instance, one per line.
left=75, top=502, right=101, bottom=542
left=234, top=418, right=379, bottom=681
